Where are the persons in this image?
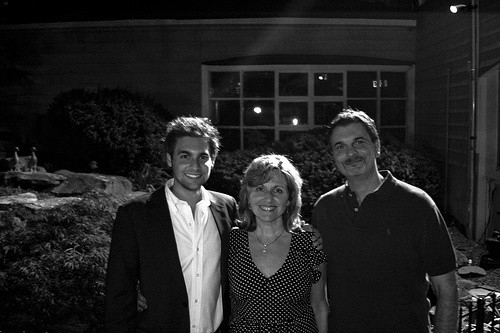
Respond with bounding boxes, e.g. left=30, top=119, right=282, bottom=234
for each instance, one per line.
left=137, top=154, right=330, bottom=333
left=234, top=112, right=460, bottom=333
left=105, top=118, right=324, bottom=333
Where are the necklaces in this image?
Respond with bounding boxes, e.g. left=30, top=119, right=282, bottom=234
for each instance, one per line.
left=252, top=227, right=285, bottom=255
